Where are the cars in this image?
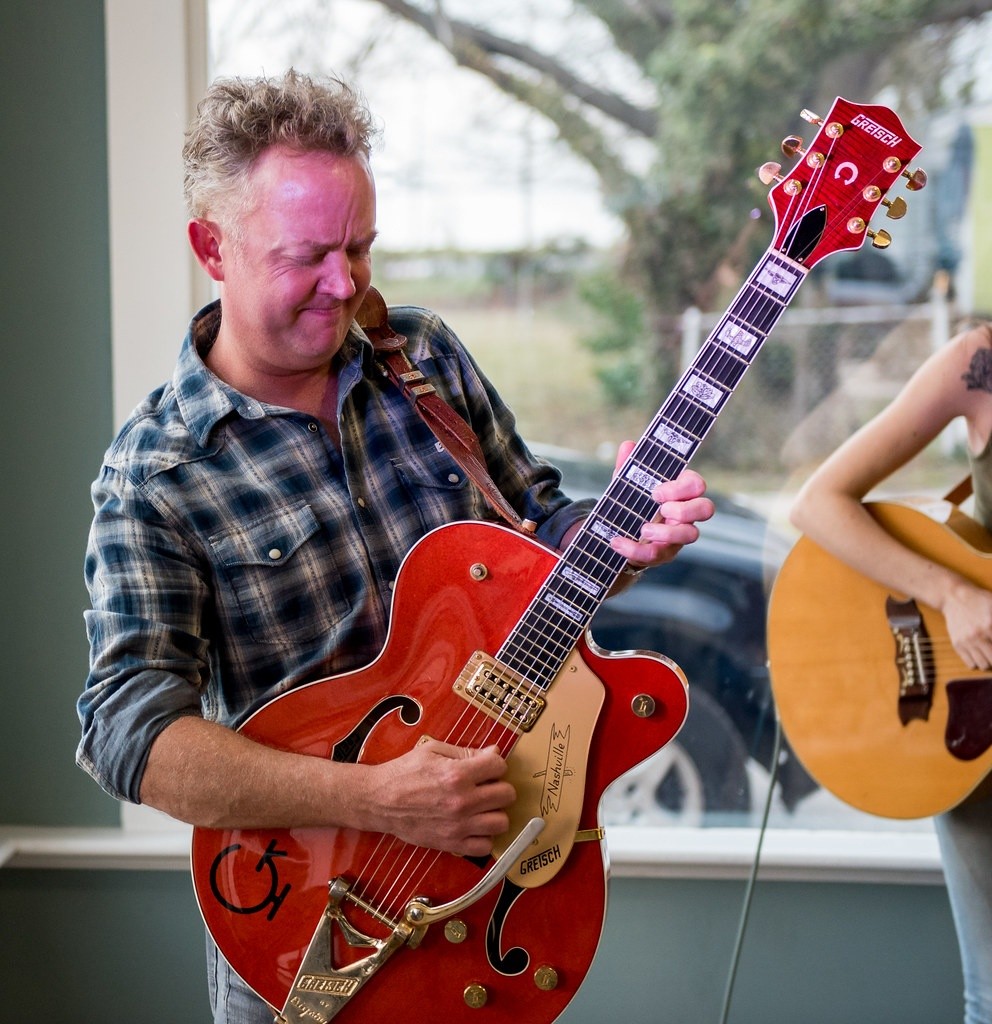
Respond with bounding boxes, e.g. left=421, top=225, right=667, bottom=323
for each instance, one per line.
left=523, top=438, right=822, bottom=827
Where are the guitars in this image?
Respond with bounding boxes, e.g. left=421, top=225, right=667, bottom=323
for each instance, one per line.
left=764, top=495, right=992, bottom=822
left=189, top=94, right=931, bottom=1024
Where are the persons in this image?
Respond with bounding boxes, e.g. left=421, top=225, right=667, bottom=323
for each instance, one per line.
left=789, top=329, right=991, bottom=1024
left=77, top=71, right=713, bottom=1024
